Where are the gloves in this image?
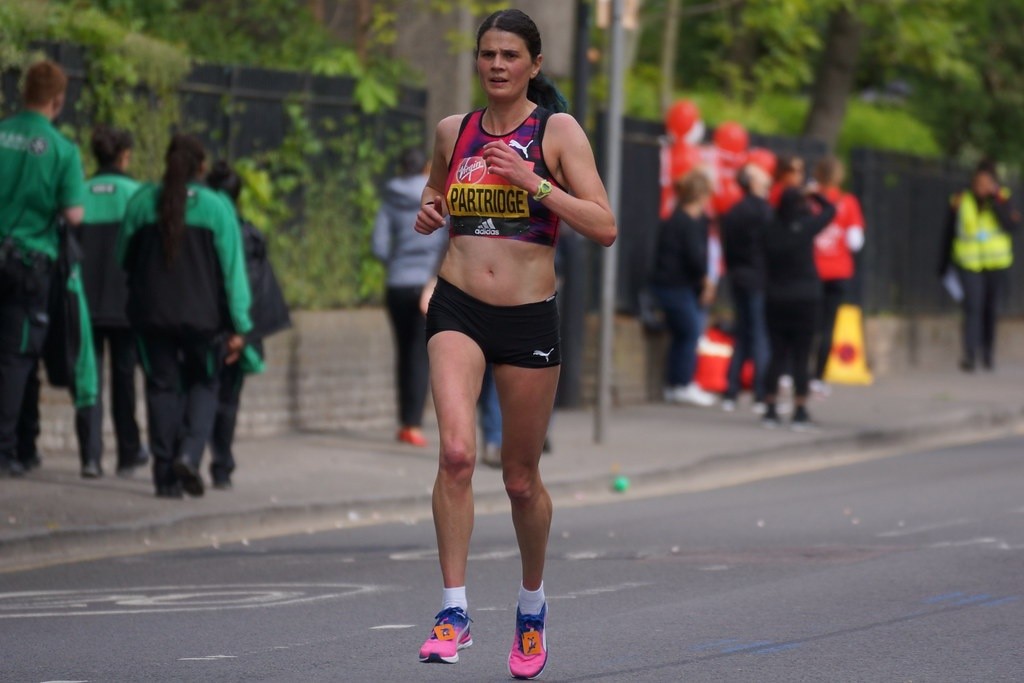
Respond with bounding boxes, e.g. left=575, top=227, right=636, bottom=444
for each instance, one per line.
left=533, top=179, right=552, bottom=201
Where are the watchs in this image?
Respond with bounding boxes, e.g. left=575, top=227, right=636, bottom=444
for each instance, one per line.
left=534, top=180, right=552, bottom=201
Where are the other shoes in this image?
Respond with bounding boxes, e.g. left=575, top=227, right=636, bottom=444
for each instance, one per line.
left=480, top=449, right=502, bottom=471
left=152, top=455, right=205, bottom=498
left=809, top=378, right=833, bottom=398
left=396, top=426, right=430, bottom=447
left=211, top=467, right=234, bottom=490
left=957, top=354, right=996, bottom=373
left=0, top=455, right=42, bottom=480
left=752, top=402, right=766, bottom=414
left=722, top=400, right=734, bottom=411
left=790, top=405, right=822, bottom=435
left=662, top=383, right=717, bottom=407
left=760, top=405, right=782, bottom=430
left=116, top=450, right=150, bottom=477
left=79, top=457, right=103, bottom=479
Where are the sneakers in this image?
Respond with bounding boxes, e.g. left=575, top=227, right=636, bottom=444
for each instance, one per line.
left=418, top=606, right=473, bottom=664
left=507, top=601, right=549, bottom=680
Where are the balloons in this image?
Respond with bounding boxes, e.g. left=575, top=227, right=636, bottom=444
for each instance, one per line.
left=748, top=149, right=776, bottom=175
left=657, top=152, right=744, bottom=218
left=668, top=101, right=704, bottom=145
left=714, top=122, right=748, bottom=157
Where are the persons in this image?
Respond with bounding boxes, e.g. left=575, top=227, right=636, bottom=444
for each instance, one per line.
left=414, top=8, right=618, bottom=679
left=419, top=251, right=563, bottom=469
left=204, top=160, right=289, bottom=488
left=74, top=125, right=150, bottom=477
left=0, top=63, right=85, bottom=475
left=934, top=158, right=1024, bottom=374
left=111, top=136, right=252, bottom=498
left=653, top=170, right=716, bottom=405
left=372, top=147, right=450, bottom=447
left=717, top=155, right=866, bottom=430
